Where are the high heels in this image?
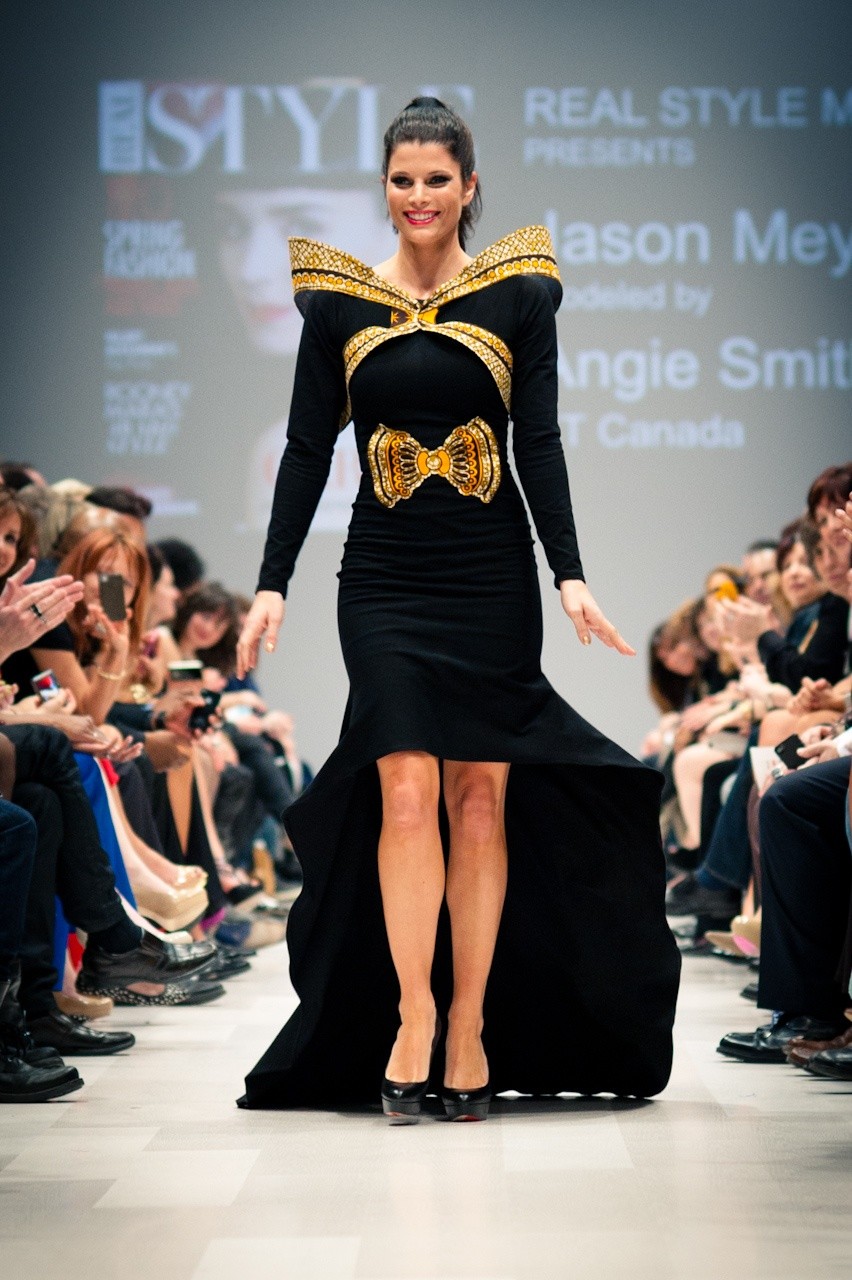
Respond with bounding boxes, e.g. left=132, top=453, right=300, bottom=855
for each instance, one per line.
left=379, top=1011, right=446, bottom=1118
left=437, top=1063, right=494, bottom=1123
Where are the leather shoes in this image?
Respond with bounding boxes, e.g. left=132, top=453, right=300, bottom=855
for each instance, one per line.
left=1, top=875, right=291, bottom=1102
left=663, top=845, right=852, bottom=1080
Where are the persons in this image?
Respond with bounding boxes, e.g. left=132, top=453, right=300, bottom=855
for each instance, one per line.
left=0, top=458, right=324, bottom=1114
left=228, top=87, right=680, bottom=1121
left=633, top=458, right=852, bottom=1089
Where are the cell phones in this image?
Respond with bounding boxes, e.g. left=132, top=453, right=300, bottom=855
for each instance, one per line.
left=31, top=670, right=60, bottom=703
left=774, top=734, right=808, bottom=770
left=189, top=689, right=222, bottom=731
left=97, top=572, right=126, bottom=623
